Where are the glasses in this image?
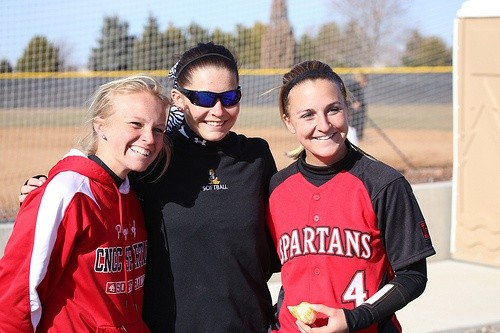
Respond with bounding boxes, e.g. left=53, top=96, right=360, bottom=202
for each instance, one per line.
left=176, top=86, right=241, bottom=108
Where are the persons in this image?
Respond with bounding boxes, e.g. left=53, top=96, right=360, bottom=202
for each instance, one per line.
left=19, top=41, right=282, bottom=333
left=0, top=75, right=171, bottom=333
left=264, top=60, right=436, bottom=333
left=344, top=73, right=369, bottom=147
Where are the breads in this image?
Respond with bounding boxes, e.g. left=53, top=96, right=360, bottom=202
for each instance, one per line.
left=287, top=301, right=316, bottom=324
